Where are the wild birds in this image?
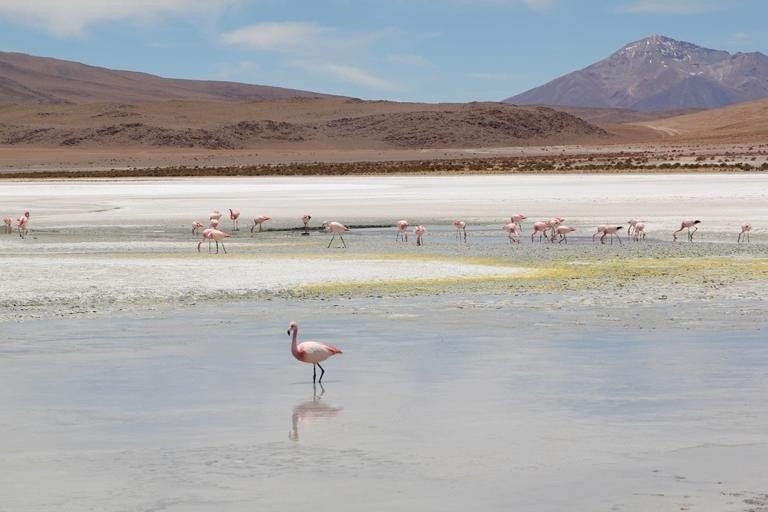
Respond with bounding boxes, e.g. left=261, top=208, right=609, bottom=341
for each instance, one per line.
left=301, top=214, right=311, bottom=234
left=672, top=219, right=701, bottom=243
left=287, top=322, right=344, bottom=382
left=502, top=214, right=577, bottom=245
left=413, top=225, right=426, bottom=246
left=287, top=383, right=344, bottom=442
left=3, top=211, right=30, bottom=239
left=452, top=219, right=467, bottom=243
left=737, top=223, right=753, bottom=244
left=321, top=220, right=350, bottom=249
left=192, top=208, right=241, bottom=255
left=592, top=220, right=647, bottom=246
left=250, top=216, right=272, bottom=235
left=396, top=220, right=409, bottom=243
